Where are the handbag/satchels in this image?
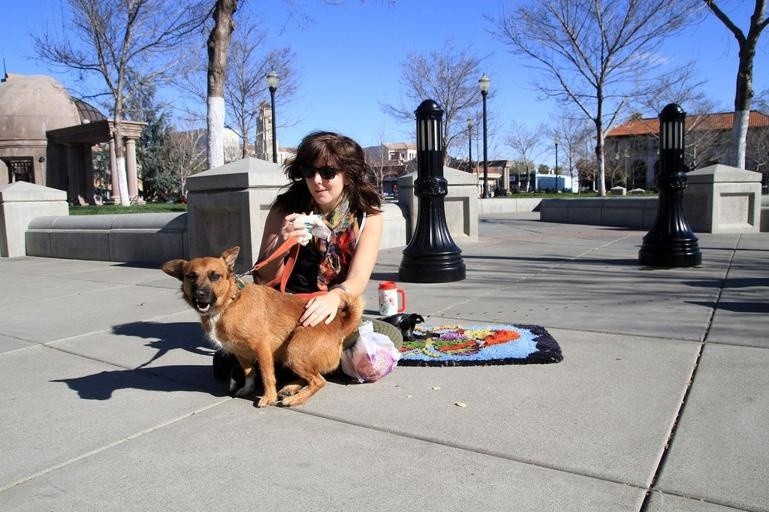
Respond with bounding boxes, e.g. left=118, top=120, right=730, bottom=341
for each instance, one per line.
left=379, top=313, right=440, bottom=341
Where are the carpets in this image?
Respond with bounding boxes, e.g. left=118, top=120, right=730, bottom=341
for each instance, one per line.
left=393, top=323, right=565, bottom=369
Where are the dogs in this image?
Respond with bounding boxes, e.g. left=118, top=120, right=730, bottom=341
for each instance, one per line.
left=161, top=244, right=364, bottom=410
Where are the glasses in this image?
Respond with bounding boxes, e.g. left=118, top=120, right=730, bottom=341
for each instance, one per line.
left=302, top=165, right=343, bottom=180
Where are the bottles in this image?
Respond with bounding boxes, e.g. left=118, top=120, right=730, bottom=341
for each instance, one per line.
left=377, top=281, right=406, bottom=316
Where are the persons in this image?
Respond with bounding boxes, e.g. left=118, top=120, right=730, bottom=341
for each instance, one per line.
left=212, top=130, right=405, bottom=396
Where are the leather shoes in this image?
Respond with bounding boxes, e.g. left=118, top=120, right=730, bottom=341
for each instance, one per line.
left=225, top=365, right=243, bottom=393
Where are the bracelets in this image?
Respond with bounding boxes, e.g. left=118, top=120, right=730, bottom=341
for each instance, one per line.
left=328, top=282, right=350, bottom=296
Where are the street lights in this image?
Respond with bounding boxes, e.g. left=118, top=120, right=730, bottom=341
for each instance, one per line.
left=266, top=65, right=280, bottom=163
left=554, top=136, right=559, bottom=194
left=467, top=115, right=474, bottom=172
left=479, top=71, right=492, bottom=199
